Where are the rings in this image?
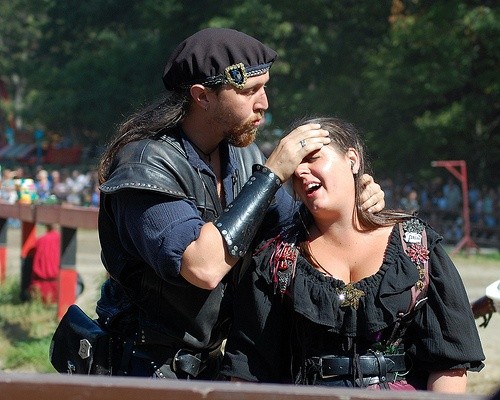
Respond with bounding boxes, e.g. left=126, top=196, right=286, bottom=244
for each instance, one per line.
left=300, top=140, right=306, bottom=147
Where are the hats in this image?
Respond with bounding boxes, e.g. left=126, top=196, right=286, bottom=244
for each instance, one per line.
left=162, top=27, right=278, bottom=93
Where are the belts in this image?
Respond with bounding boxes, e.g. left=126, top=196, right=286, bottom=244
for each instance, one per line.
left=306, top=354, right=414, bottom=379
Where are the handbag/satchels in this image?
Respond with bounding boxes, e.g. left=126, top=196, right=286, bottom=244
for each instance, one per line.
left=49, top=305, right=113, bottom=378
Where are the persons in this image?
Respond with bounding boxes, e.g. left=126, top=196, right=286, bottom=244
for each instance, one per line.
left=0, top=167, right=99, bottom=206
left=379, top=172, right=500, bottom=245
left=217, top=117, right=486, bottom=392
left=49, top=27, right=385, bottom=380
